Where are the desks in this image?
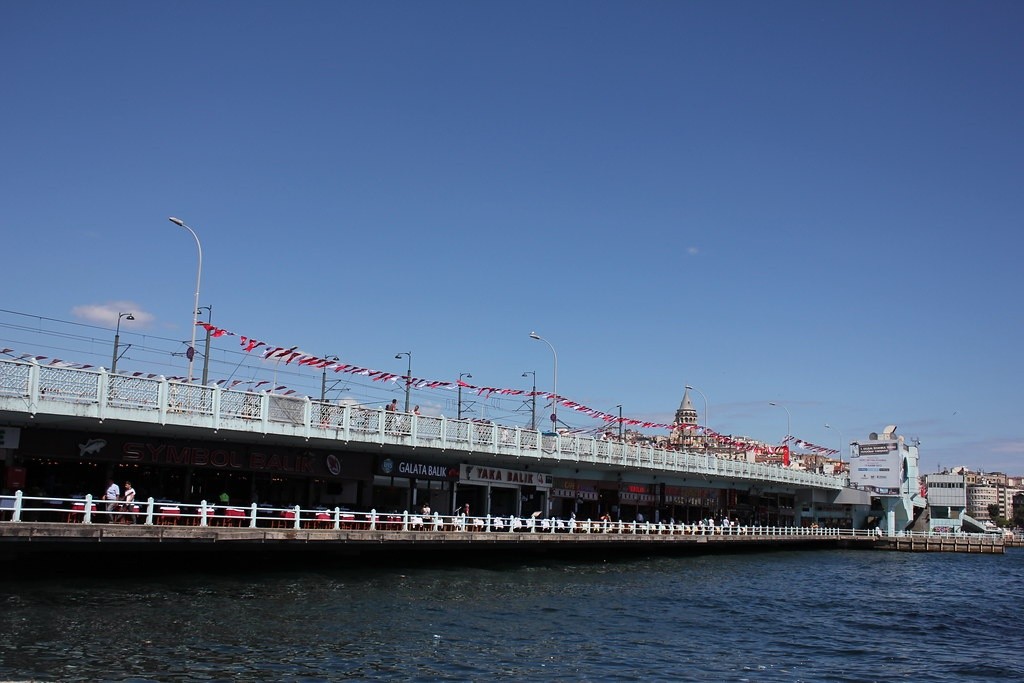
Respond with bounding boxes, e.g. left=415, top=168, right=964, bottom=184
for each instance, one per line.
left=114, top=504, right=139, bottom=524
left=67, top=503, right=97, bottom=523
left=223, top=509, right=245, bottom=527
left=192, top=508, right=215, bottom=526
left=271, top=510, right=401, bottom=531
left=156, top=506, right=180, bottom=525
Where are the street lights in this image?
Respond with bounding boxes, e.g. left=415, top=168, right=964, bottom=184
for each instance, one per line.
left=522, top=371, right=536, bottom=432
left=686, top=386, right=708, bottom=455
left=457, top=373, right=472, bottom=441
left=193, top=305, right=211, bottom=413
left=108, top=312, right=135, bottom=406
left=320, top=354, right=339, bottom=422
left=530, top=334, right=560, bottom=433
left=168, top=216, right=201, bottom=415
left=395, top=352, right=411, bottom=416
left=769, top=402, right=790, bottom=456
left=825, top=424, right=842, bottom=469
left=617, top=404, right=624, bottom=445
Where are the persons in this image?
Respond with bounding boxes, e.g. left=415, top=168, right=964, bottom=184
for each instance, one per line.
left=700, top=443, right=711, bottom=456
left=419, top=502, right=431, bottom=531
left=463, top=503, right=470, bottom=523
left=939, top=527, right=949, bottom=532
left=319, top=399, right=330, bottom=429
left=600, top=432, right=681, bottom=451
left=584, top=513, right=591, bottom=521
left=776, top=460, right=782, bottom=467
left=385, top=399, right=420, bottom=434
left=378, top=502, right=387, bottom=512
left=247, top=491, right=258, bottom=507
left=102, top=477, right=136, bottom=524
left=601, top=512, right=611, bottom=527
